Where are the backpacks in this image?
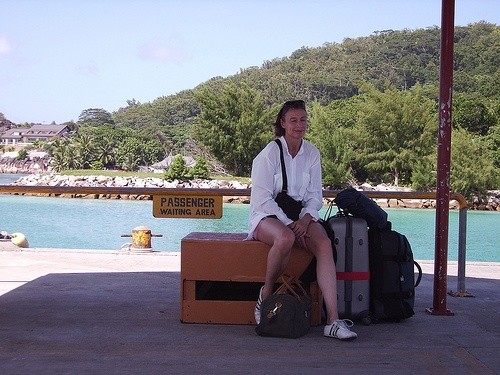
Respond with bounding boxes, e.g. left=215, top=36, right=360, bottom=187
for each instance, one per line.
left=368, top=221, right=422, bottom=323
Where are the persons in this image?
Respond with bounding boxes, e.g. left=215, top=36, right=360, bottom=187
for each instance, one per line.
left=244, top=100, right=358, bottom=339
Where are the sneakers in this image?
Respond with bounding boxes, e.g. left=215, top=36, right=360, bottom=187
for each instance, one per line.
left=324, top=319, right=358, bottom=341
left=255, top=285, right=264, bottom=325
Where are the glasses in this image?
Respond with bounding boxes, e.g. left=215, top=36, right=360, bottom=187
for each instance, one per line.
left=283, top=100, right=305, bottom=107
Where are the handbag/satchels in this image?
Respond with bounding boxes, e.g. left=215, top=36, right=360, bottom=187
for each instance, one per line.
left=255, top=272, right=312, bottom=338
left=336, top=188, right=388, bottom=230
left=268, top=193, right=303, bottom=223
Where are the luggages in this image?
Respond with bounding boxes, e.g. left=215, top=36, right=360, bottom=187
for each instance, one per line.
left=329, top=208, right=371, bottom=326
